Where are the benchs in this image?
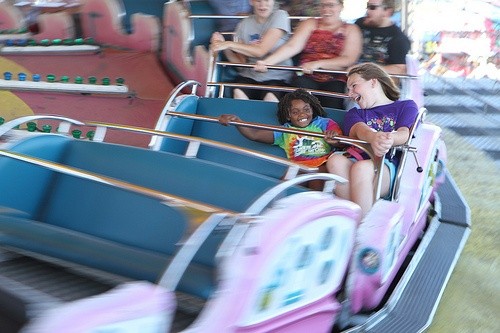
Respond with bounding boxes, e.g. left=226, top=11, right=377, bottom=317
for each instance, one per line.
left=1, top=0, right=444, bottom=332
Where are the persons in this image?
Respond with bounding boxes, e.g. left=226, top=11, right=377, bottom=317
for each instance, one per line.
left=219, top=90, right=343, bottom=191
left=326, top=61, right=419, bottom=214
left=210, top=0, right=296, bottom=103
left=353, top=0, right=411, bottom=90
left=255, top=0, right=363, bottom=112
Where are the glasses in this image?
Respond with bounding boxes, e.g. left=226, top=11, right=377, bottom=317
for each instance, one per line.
left=367, top=2, right=385, bottom=10
